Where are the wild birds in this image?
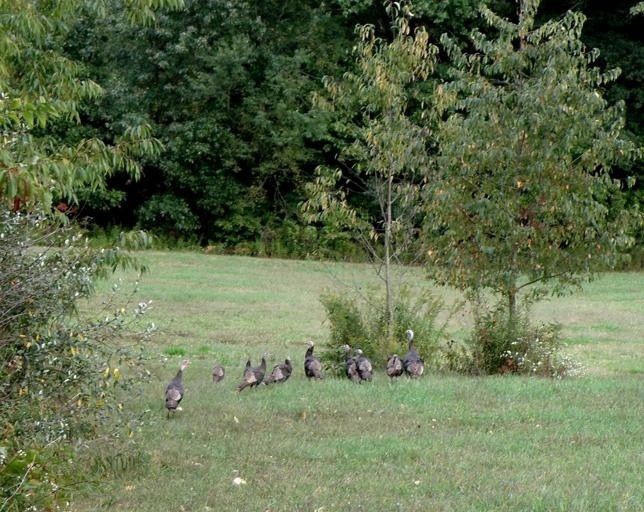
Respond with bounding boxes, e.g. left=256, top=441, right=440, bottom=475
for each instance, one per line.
left=262, top=356, right=292, bottom=386
left=387, top=354, right=404, bottom=384
left=212, top=364, right=225, bottom=383
left=341, top=344, right=373, bottom=384
left=304, top=341, right=324, bottom=382
left=165, top=358, right=192, bottom=419
left=236, top=353, right=267, bottom=393
left=403, top=330, right=424, bottom=384
